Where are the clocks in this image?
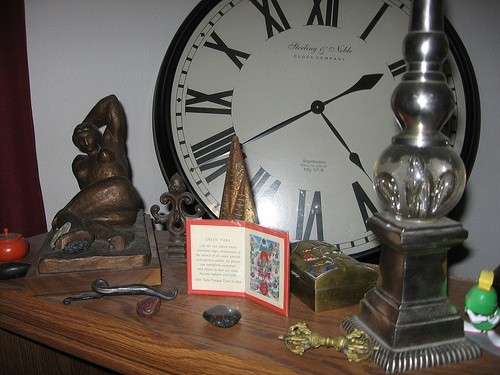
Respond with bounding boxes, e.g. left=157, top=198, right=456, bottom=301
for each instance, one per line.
left=152, top=0, right=483, bottom=269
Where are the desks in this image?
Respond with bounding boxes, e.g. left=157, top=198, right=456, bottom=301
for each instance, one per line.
left=1, top=230, right=500, bottom=375
